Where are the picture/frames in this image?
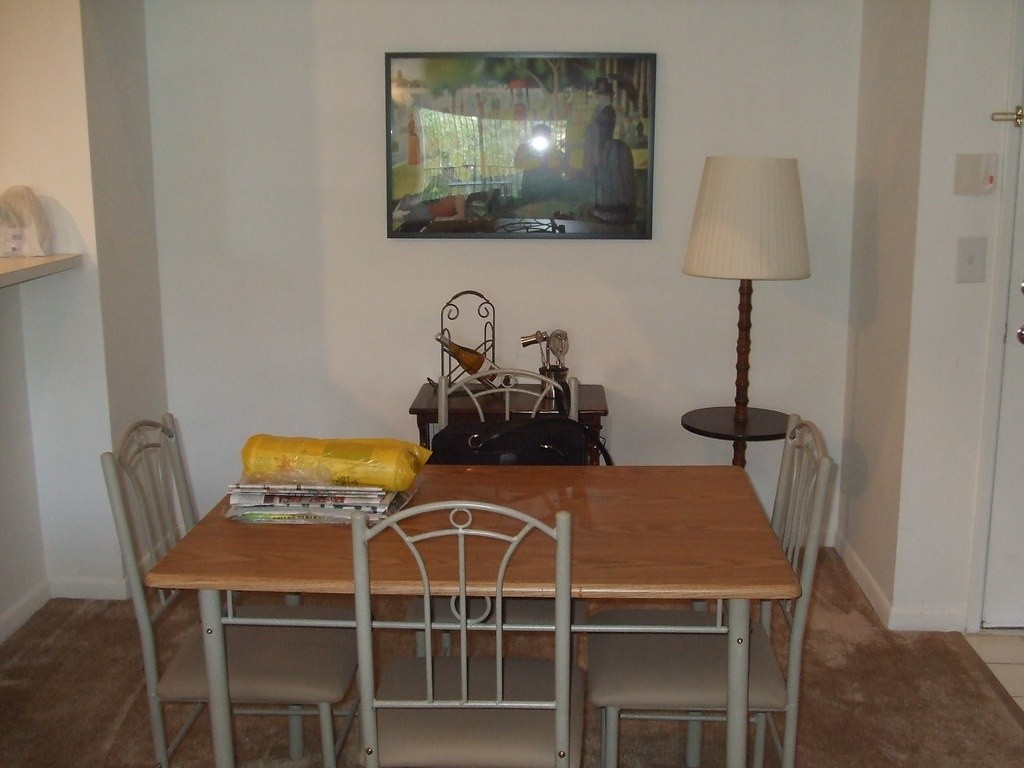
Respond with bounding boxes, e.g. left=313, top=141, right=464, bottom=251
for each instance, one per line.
left=385, top=52, right=656, bottom=240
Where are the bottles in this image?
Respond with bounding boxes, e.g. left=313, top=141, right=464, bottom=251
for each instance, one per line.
left=435, top=332, right=511, bottom=393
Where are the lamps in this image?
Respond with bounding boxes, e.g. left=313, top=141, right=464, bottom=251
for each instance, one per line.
left=681, top=153, right=810, bottom=470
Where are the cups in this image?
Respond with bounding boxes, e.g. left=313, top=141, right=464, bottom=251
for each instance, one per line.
left=539, top=366, right=569, bottom=399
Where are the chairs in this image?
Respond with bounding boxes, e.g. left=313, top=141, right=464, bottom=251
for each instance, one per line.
left=350, top=499, right=586, bottom=768
left=100, top=413, right=372, bottom=768
left=438, top=369, right=582, bottom=432
left=587, top=413, right=832, bottom=767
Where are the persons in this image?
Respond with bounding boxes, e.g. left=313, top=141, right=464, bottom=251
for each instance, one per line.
left=514, top=106, right=637, bottom=209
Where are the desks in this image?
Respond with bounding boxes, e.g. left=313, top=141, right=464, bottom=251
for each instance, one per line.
left=403, top=384, right=608, bottom=658
left=145, top=465, right=804, bottom=768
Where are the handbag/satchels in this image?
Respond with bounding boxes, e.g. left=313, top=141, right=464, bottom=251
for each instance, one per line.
left=426, top=413, right=614, bottom=467
